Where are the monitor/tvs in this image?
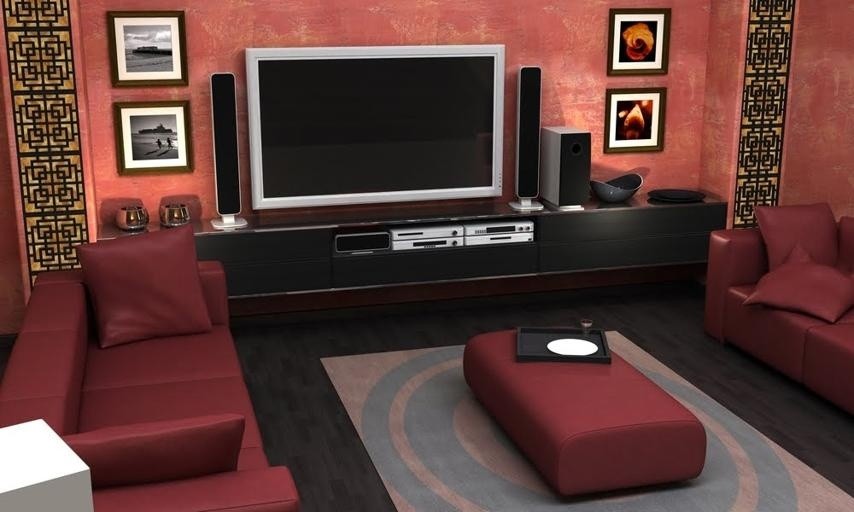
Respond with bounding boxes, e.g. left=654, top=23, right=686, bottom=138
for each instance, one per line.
left=244, top=43, right=506, bottom=211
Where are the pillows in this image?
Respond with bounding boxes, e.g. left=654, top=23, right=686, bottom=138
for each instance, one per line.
left=744, top=241, right=853, bottom=322
left=78, top=221, right=214, bottom=347
left=57, top=413, right=247, bottom=491
left=756, top=202, right=841, bottom=270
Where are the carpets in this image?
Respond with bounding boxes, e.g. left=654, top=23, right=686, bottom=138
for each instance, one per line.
left=320, top=325, right=854, bottom=512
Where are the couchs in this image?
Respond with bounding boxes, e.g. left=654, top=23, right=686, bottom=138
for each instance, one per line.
left=1, top=261, right=299, bottom=511
left=707, top=217, right=853, bottom=415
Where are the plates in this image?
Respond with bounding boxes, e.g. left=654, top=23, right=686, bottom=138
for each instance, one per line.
left=648, top=189, right=706, bottom=202
left=547, top=338, right=599, bottom=355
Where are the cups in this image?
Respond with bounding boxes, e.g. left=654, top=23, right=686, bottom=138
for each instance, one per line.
left=580, top=319, right=593, bottom=334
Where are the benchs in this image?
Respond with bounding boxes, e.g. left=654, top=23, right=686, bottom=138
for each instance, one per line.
left=464, top=330, right=707, bottom=494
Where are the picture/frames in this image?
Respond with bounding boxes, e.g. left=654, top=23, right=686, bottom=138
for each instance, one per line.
left=606, top=6, right=669, bottom=76
left=111, top=100, right=194, bottom=175
left=603, top=89, right=666, bottom=154
left=105, top=11, right=187, bottom=87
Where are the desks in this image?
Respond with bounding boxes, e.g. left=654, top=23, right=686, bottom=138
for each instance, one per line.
left=0, top=420, right=94, bottom=512
left=194, top=189, right=731, bottom=316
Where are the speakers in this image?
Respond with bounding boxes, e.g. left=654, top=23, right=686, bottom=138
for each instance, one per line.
left=208, top=72, right=249, bottom=230
left=508, top=64, right=545, bottom=212
left=540, top=126, right=591, bottom=211
